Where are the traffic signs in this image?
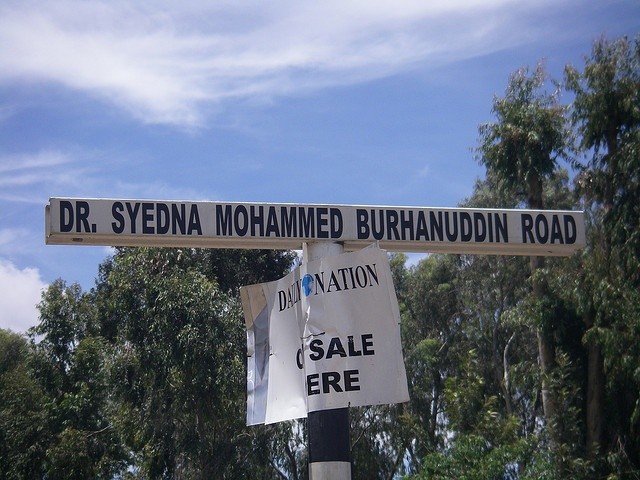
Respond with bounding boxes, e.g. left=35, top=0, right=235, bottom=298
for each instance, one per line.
left=43, top=196, right=588, bottom=254
